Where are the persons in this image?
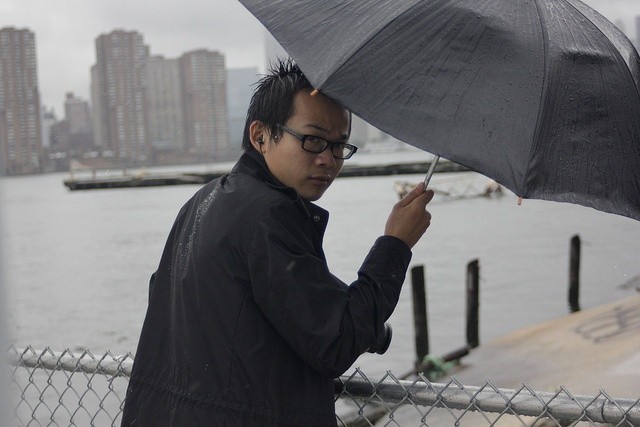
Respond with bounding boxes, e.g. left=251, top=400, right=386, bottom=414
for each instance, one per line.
left=120, top=57, right=435, bottom=427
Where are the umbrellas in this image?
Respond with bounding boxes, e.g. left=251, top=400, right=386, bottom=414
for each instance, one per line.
left=234, top=0, right=639, bottom=223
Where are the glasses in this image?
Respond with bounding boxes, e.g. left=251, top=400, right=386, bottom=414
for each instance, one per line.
left=273, top=121, right=359, bottom=160
